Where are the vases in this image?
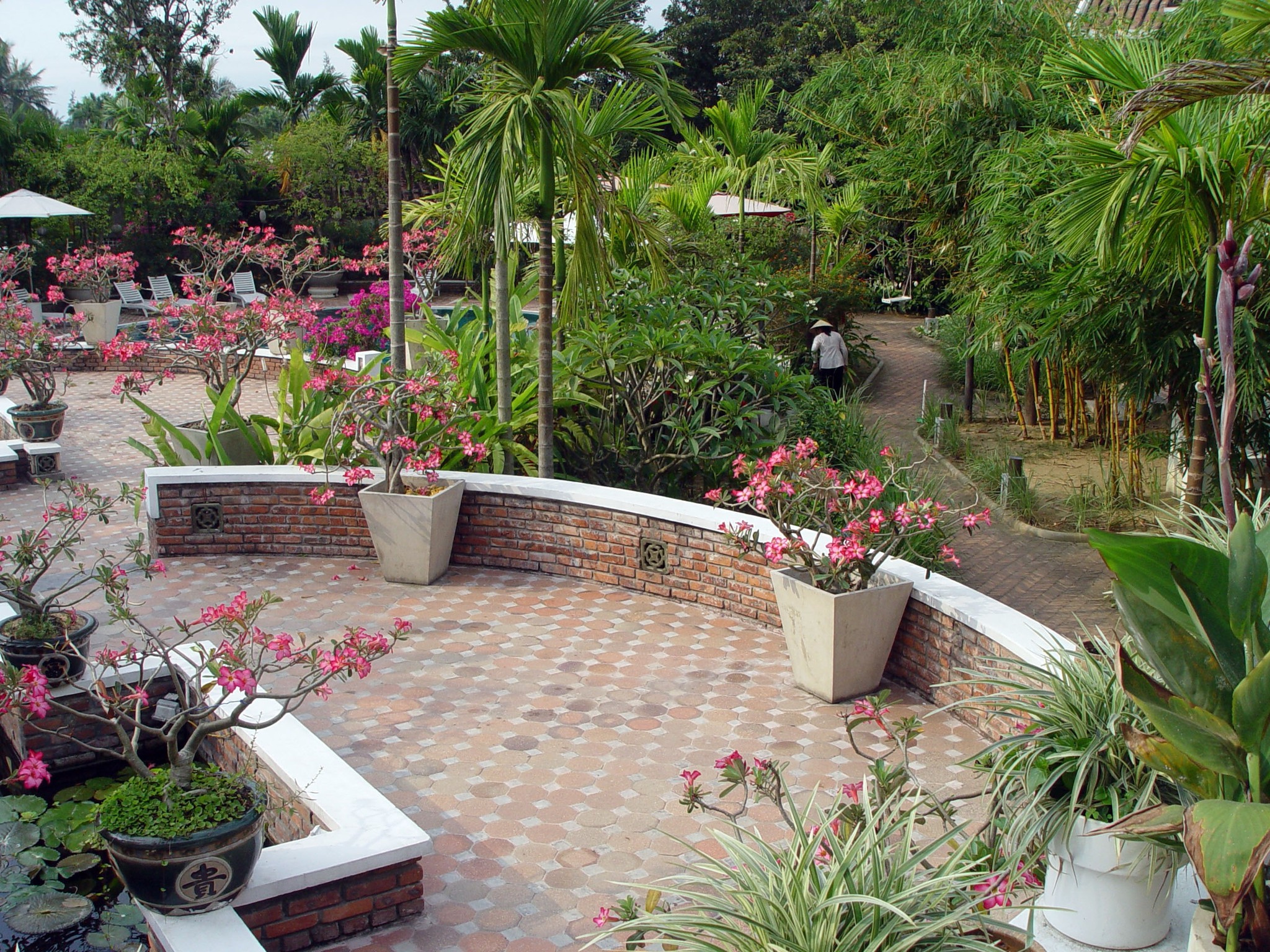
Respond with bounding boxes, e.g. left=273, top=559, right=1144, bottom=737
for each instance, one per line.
left=23, top=300, right=43, bottom=328
left=265, top=309, right=307, bottom=355
left=0, top=609, right=100, bottom=689
left=95, top=769, right=269, bottom=921
left=403, top=315, right=450, bottom=371
left=168, top=417, right=265, bottom=466
left=763, top=560, right=915, bottom=705
left=210, top=301, right=237, bottom=315
left=6, top=401, right=68, bottom=443
left=61, top=285, right=111, bottom=300
left=357, top=473, right=466, bottom=586
left=72, top=299, right=122, bottom=346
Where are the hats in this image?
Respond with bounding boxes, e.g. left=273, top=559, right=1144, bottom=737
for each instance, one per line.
left=809, top=318, right=834, bottom=334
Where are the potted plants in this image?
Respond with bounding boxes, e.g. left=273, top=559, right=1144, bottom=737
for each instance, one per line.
left=285, top=194, right=346, bottom=298
left=921, top=608, right=1206, bottom=950
left=571, top=767, right=1046, bottom=952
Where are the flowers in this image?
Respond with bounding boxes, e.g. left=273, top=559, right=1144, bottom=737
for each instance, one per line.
left=589, top=687, right=1048, bottom=952
left=0, top=220, right=494, bottom=797
left=704, top=434, right=993, bottom=590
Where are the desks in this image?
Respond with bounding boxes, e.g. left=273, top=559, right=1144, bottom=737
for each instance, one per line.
left=172, top=271, right=204, bottom=277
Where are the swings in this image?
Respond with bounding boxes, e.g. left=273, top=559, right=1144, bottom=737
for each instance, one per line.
left=880, top=218, right=917, bottom=304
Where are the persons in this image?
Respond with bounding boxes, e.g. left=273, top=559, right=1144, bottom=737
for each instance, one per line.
left=811, top=318, right=848, bottom=393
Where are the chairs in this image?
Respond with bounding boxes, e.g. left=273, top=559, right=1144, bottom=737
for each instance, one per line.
left=9, top=286, right=73, bottom=330
left=410, top=269, right=440, bottom=302
left=229, top=270, right=268, bottom=309
left=147, top=274, right=195, bottom=306
left=113, top=280, right=165, bottom=318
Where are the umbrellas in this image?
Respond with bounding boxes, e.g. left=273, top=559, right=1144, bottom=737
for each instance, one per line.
left=0, top=187, right=97, bottom=301
